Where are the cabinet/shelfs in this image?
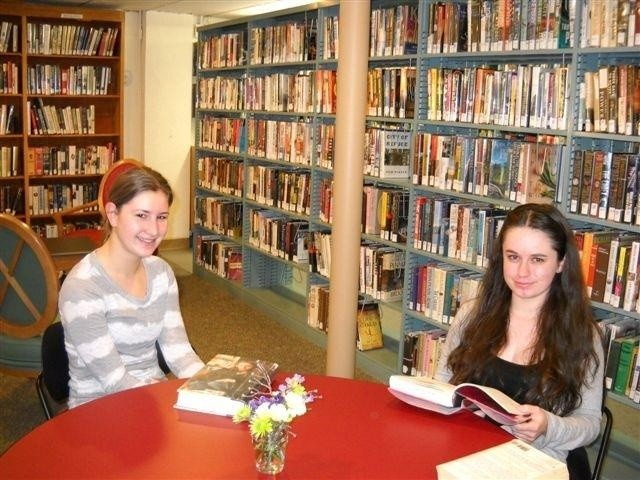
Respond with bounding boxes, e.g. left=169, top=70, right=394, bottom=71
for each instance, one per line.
left=25, top=5, right=123, bottom=238
left=1, top=1, right=26, bottom=226
left=354, top=0, right=640, bottom=414
left=190, top=0, right=339, bottom=346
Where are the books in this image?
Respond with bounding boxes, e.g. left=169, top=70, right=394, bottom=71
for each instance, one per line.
left=356, top=0, right=640, bottom=404
left=0, top=12, right=119, bottom=237
left=194, top=17, right=340, bottom=335
left=387, top=373, right=534, bottom=426
left=435, top=438, right=571, bottom=480
left=172, top=352, right=280, bottom=422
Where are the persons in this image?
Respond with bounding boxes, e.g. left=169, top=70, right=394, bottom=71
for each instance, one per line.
left=59, top=166, right=207, bottom=412
left=429, top=203, right=605, bottom=459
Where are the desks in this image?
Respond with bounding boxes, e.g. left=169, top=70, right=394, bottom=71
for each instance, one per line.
left=0, top=375, right=572, bottom=480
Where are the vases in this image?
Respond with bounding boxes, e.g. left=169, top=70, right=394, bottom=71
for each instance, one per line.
left=254, top=425, right=286, bottom=474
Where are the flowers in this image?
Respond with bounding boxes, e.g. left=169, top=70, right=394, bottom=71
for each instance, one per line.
left=233, top=360, right=320, bottom=463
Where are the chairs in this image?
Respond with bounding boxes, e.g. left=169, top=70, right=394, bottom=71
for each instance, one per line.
left=1, top=213, right=67, bottom=370
left=567, top=407, right=615, bottom=480
left=35, top=321, right=71, bottom=422
